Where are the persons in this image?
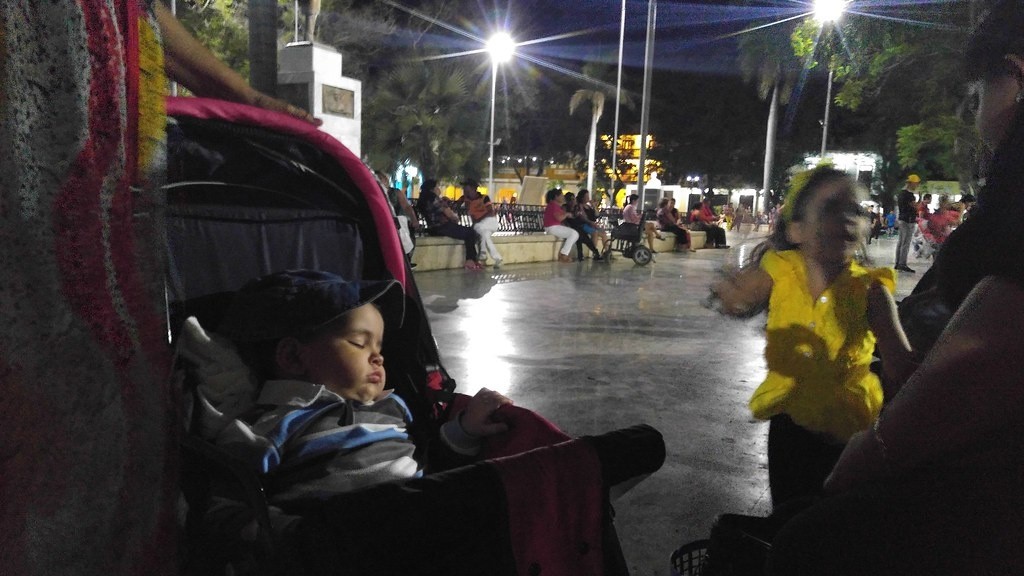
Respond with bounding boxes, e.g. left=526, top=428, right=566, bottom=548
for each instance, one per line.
left=705, top=165, right=917, bottom=515
left=375, top=170, right=421, bottom=267
left=541, top=188, right=579, bottom=263
left=865, top=181, right=976, bottom=277
left=621, top=194, right=665, bottom=253
left=416, top=180, right=482, bottom=269
left=822, top=0, right=1024, bottom=576
left=655, top=197, right=696, bottom=253
left=560, top=192, right=600, bottom=261
left=237, top=268, right=511, bottom=515
left=687, top=198, right=731, bottom=249
left=576, top=189, right=610, bottom=252
left=455, top=179, right=503, bottom=269
left=716, top=202, right=778, bottom=233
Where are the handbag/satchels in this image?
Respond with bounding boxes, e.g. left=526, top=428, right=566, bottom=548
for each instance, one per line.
left=583, top=223, right=596, bottom=233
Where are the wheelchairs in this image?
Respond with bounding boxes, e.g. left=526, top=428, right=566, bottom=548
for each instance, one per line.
left=600, top=211, right=656, bottom=266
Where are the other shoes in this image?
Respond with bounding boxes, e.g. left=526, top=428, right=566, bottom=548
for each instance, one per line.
left=651, top=250, right=659, bottom=253
left=465, top=262, right=486, bottom=272
left=895, top=264, right=915, bottom=274
left=559, top=255, right=573, bottom=262
left=656, top=235, right=665, bottom=241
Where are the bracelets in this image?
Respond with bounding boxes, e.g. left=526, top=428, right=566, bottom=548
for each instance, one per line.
left=871, top=414, right=895, bottom=456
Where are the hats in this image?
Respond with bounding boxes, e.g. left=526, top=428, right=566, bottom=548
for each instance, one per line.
left=217, top=270, right=405, bottom=340
left=906, top=175, right=921, bottom=183
left=460, top=178, right=479, bottom=191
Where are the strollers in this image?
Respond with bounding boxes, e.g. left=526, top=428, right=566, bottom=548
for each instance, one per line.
left=166, top=97, right=665, bottom=576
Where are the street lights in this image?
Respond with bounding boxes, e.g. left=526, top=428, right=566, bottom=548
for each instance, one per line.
left=814, top=0, right=847, bottom=158
left=486, top=31, right=516, bottom=209
